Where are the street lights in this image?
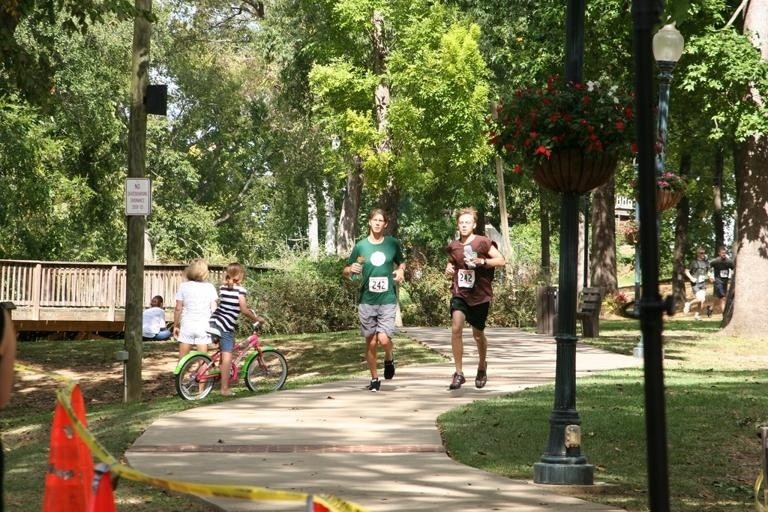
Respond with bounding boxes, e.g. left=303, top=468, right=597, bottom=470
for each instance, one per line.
left=631, top=22, right=686, bottom=360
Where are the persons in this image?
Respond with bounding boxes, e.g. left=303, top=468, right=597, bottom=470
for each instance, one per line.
left=172, top=259, right=218, bottom=396
left=341, top=209, right=407, bottom=391
left=204, top=262, right=265, bottom=396
left=445, top=206, right=507, bottom=388
left=706, top=246, right=734, bottom=317
left=143, top=296, right=171, bottom=340
left=683, top=247, right=709, bottom=321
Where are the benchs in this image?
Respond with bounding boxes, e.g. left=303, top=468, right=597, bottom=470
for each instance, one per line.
left=574, top=287, right=604, bottom=337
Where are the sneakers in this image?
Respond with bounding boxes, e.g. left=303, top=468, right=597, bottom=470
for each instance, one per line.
left=368, top=378, right=380, bottom=392
left=384, top=353, right=394, bottom=379
left=475, top=362, right=487, bottom=387
left=450, top=372, right=465, bottom=389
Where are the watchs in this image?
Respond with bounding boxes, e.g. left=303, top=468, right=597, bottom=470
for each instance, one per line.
left=480, top=257, right=487, bottom=266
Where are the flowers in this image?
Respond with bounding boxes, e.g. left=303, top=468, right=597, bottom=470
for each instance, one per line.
left=484, top=73, right=639, bottom=174
left=630, top=172, right=689, bottom=198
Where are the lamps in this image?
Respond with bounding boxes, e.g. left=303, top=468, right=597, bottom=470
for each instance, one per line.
left=142, top=85, right=166, bottom=115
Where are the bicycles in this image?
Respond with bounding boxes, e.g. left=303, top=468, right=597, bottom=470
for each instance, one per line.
left=173, top=309, right=288, bottom=401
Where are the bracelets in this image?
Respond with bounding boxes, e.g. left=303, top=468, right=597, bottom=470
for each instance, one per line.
left=401, top=267, right=406, bottom=273
